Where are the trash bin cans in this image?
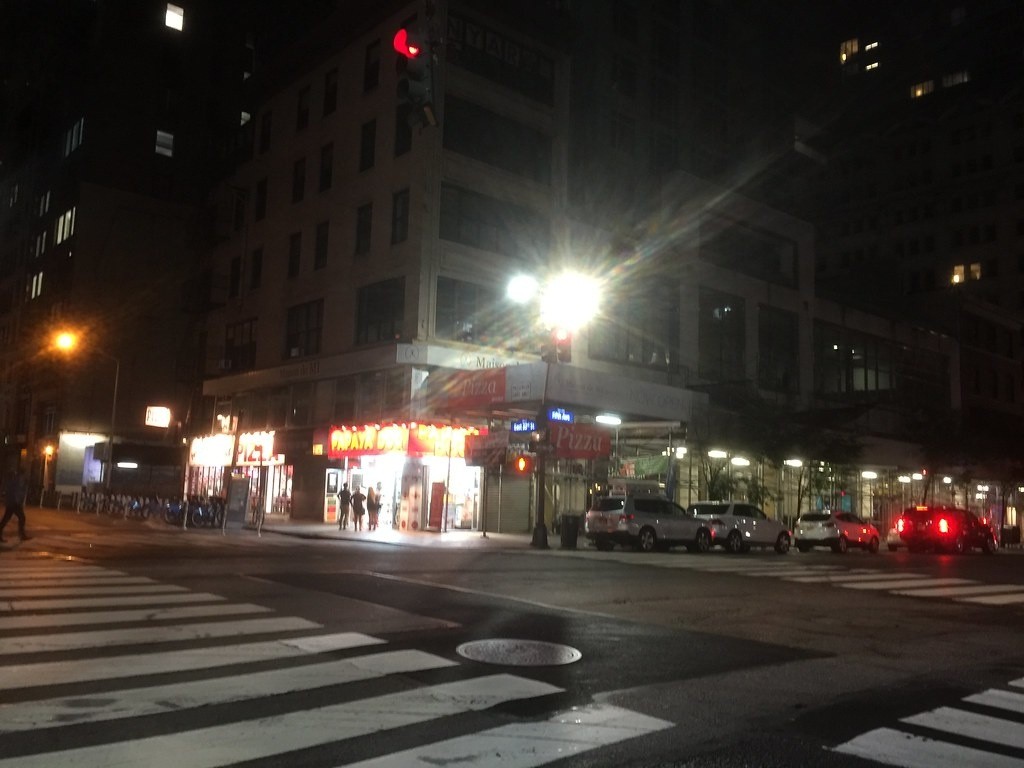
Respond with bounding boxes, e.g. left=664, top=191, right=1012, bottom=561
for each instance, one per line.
left=560, top=512, right=580, bottom=549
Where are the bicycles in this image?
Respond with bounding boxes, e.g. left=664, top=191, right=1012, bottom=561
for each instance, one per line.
left=68, top=491, right=226, bottom=531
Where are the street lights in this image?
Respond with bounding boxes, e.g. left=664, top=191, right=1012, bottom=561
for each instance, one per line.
left=54, top=333, right=122, bottom=484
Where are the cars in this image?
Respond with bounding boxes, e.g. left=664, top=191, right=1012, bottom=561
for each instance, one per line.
left=887, top=525, right=913, bottom=552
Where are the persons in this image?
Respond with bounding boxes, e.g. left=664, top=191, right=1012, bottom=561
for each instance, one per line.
left=349, top=486, right=366, bottom=532
left=337, top=483, right=352, bottom=530
left=376, top=482, right=384, bottom=515
left=0, top=470, right=33, bottom=542
left=367, top=487, right=379, bottom=530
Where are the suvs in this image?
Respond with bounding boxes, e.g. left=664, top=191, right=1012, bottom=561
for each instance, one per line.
left=794, top=510, right=882, bottom=555
left=584, top=493, right=715, bottom=553
left=686, top=501, right=793, bottom=555
left=900, top=506, right=1000, bottom=554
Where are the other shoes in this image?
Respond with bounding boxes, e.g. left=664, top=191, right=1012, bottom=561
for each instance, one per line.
left=0, top=535, right=9, bottom=543
left=19, top=534, right=33, bottom=542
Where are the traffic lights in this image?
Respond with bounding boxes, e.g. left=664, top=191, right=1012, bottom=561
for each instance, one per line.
left=540, top=329, right=556, bottom=363
left=516, top=455, right=532, bottom=472
left=557, top=329, right=573, bottom=361
